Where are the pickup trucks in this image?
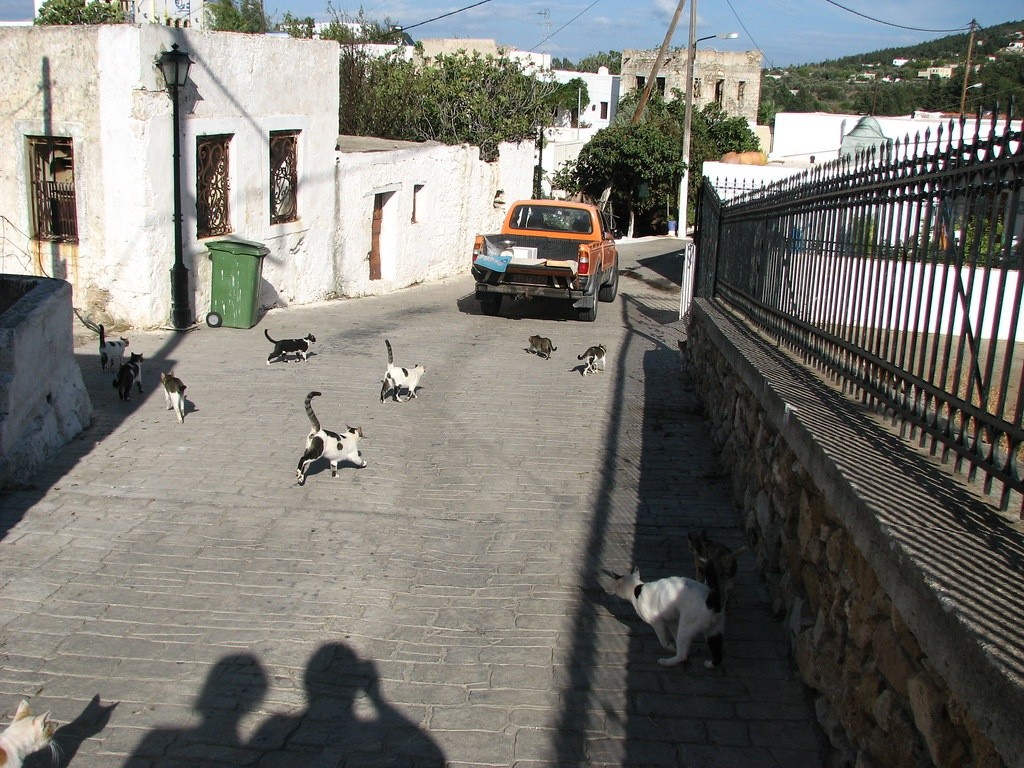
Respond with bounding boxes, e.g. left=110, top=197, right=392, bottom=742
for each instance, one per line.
left=470, top=199, right=619, bottom=321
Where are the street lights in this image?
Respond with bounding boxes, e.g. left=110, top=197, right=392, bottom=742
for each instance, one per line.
left=677, top=32, right=737, bottom=240
left=958, top=81, right=983, bottom=116
left=155, top=42, right=197, bottom=329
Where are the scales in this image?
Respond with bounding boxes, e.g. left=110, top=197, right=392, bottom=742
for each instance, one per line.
left=499, top=240, right=517, bottom=258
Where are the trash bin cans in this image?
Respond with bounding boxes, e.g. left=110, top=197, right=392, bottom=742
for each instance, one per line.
left=204, top=239, right=271, bottom=330
left=667, top=220, right=677, bottom=236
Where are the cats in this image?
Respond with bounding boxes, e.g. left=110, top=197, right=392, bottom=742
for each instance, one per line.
left=577, top=343, right=608, bottom=376
left=0, top=698, right=59, bottom=768
left=527, top=334, right=558, bottom=360
left=97, top=323, right=130, bottom=374
left=611, top=527, right=748, bottom=670
left=112, top=351, right=145, bottom=402
left=295, top=391, right=369, bottom=487
left=263, top=328, right=317, bottom=365
left=380, top=339, right=426, bottom=404
left=161, top=371, right=187, bottom=424
